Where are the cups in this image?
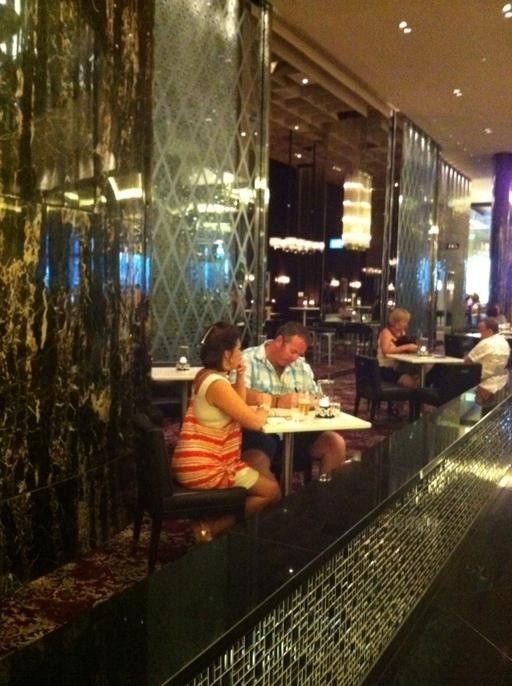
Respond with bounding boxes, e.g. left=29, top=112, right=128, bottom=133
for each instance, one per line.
left=297, top=392, right=310, bottom=416
left=331, top=397, right=342, bottom=416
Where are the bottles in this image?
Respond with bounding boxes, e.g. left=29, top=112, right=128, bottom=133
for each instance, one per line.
left=175, top=346, right=190, bottom=372
left=416, top=337, right=429, bottom=357
left=315, top=379, right=338, bottom=419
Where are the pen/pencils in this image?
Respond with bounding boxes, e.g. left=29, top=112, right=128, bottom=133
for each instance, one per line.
left=295, top=387, right=298, bottom=393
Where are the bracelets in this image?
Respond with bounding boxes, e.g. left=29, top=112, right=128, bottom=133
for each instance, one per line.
left=271, top=393, right=281, bottom=409
left=258, top=402, right=271, bottom=412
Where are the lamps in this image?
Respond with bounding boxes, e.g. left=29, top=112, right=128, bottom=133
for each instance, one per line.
left=341, top=167, right=373, bottom=251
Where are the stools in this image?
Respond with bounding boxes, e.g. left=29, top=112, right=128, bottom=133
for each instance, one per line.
left=316, top=326, right=336, bottom=367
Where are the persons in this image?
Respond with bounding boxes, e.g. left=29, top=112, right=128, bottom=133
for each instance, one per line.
left=424, top=317, right=511, bottom=402
left=465, top=292, right=508, bottom=326
left=220, top=318, right=347, bottom=481
left=376, top=307, right=434, bottom=421
left=474, top=371, right=509, bottom=406
left=168, top=320, right=281, bottom=545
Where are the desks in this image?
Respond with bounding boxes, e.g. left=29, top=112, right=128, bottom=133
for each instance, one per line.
left=289, top=306, right=320, bottom=326
